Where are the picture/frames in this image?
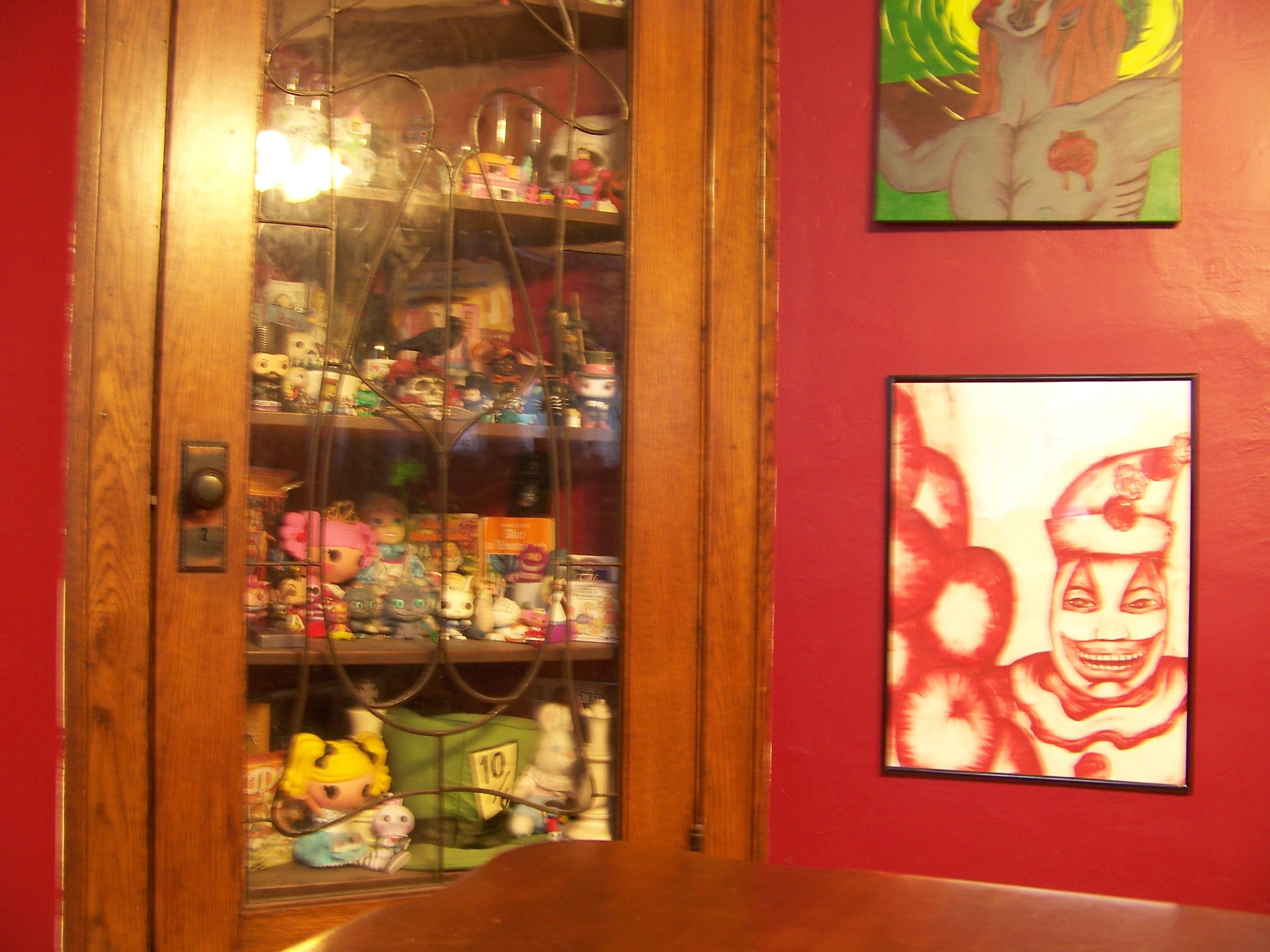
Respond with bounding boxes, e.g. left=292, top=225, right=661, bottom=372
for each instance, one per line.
left=881, top=372, right=1196, bottom=793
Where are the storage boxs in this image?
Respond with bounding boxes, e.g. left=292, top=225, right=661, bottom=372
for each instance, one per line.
left=407, top=514, right=620, bottom=642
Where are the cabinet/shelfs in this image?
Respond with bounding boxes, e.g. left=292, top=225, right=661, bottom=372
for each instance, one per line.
left=152, top=1, right=709, bottom=952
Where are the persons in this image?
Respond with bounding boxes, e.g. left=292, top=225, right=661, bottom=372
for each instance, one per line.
left=282, top=733, right=411, bottom=874
left=284, top=367, right=312, bottom=413
left=280, top=511, right=377, bottom=638
left=252, top=323, right=289, bottom=401
left=354, top=491, right=428, bottom=586
